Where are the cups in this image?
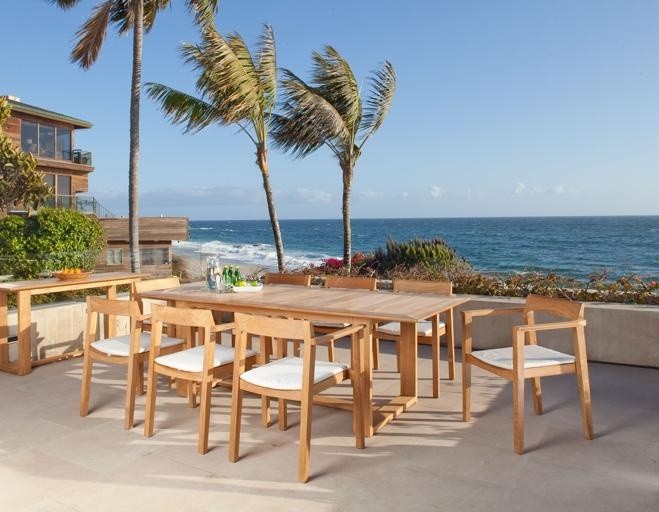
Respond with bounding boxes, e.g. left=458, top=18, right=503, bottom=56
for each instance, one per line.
left=206, top=256, right=222, bottom=288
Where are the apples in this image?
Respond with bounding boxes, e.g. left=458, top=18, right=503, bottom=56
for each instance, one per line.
left=240, top=281, right=247, bottom=287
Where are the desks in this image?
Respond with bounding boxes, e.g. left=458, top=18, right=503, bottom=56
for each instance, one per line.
left=0, top=271, right=141, bottom=377
left=131, top=281, right=471, bottom=438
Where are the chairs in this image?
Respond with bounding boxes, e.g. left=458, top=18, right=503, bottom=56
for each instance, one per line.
left=311, top=278, right=379, bottom=371
left=144, top=302, right=257, bottom=454
left=263, top=273, right=311, bottom=288
left=132, top=276, right=180, bottom=334
left=80, top=295, right=186, bottom=430
left=460, top=294, right=595, bottom=455
left=372, top=278, right=455, bottom=398
left=228, top=311, right=366, bottom=483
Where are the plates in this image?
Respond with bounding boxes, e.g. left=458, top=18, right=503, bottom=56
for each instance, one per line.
left=52, top=270, right=92, bottom=281
left=233, top=281, right=263, bottom=293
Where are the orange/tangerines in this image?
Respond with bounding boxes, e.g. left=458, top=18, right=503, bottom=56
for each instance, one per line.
left=251, top=281, right=258, bottom=286
left=235, top=281, right=239, bottom=286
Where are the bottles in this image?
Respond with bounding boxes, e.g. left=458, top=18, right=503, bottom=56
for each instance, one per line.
left=222, top=265, right=241, bottom=291
